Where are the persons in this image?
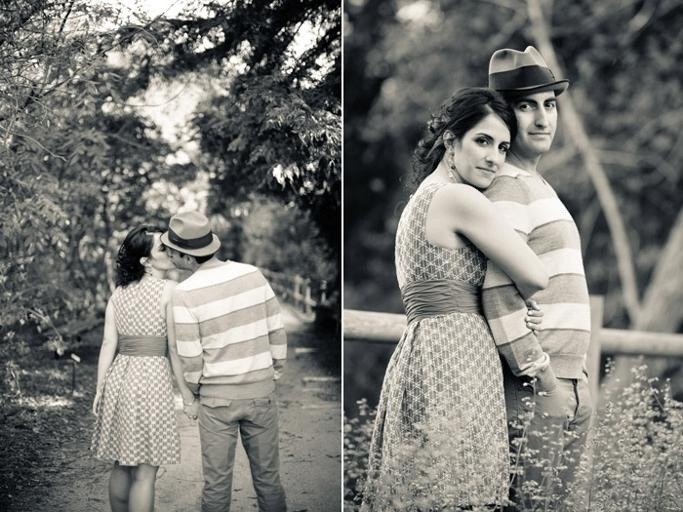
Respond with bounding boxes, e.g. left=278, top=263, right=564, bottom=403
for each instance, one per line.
left=159, top=211, right=287, bottom=512
left=92, top=224, right=200, bottom=512
left=359, top=85, right=550, bottom=512
left=481, top=45, right=593, bottom=512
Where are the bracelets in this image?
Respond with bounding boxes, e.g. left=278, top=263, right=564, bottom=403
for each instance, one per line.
left=184, top=397, right=195, bottom=406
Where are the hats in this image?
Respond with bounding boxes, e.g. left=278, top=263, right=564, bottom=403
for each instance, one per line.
left=160, top=211, right=222, bottom=257
left=488, top=46, right=570, bottom=101
